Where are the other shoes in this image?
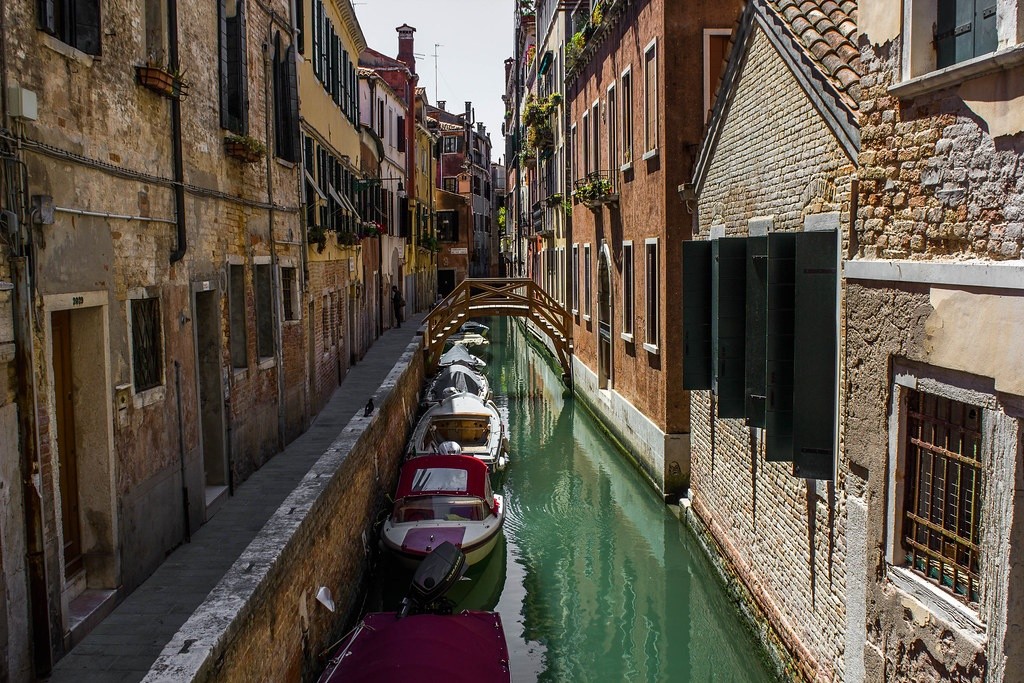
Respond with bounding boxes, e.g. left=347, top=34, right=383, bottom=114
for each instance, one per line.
left=394, top=326, right=401, bottom=328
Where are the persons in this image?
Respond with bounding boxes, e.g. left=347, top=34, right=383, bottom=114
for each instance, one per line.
left=391, top=285, right=403, bottom=329
left=436, top=294, right=444, bottom=305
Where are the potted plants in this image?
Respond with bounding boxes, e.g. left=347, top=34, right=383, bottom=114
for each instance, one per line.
left=226, top=135, right=269, bottom=162
left=337, top=230, right=361, bottom=251
left=563, top=2, right=606, bottom=72
left=518, top=94, right=562, bottom=170
left=418, top=233, right=443, bottom=256
left=137, top=52, right=189, bottom=98
left=577, top=180, right=620, bottom=209
left=306, top=225, right=328, bottom=252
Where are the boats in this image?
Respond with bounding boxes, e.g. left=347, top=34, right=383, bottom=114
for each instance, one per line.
left=460, top=321, right=489, bottom=335
left=384, top=391, right=507, bottom=570
left=316, top=541, right=512, bottom=683
left=443, top=332, right=489, bottom=357
left=422, top=359, right=492, bottom=409
left=437, top=343, right=486, bottom=370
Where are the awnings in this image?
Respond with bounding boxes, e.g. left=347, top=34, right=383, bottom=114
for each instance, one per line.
left=345, top=194, right=361, bottom=222
left=338, top=190, right=351, bottom=219
left=305, top=169, right=327, bottom=212
left=329, top=182, right=347, bottom=216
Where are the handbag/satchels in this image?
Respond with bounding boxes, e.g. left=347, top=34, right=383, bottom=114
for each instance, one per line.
left=401, top=301, right=406, bottom=307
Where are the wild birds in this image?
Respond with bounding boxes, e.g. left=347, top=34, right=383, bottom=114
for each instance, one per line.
left=363, top=398, right=375, bottom=417
